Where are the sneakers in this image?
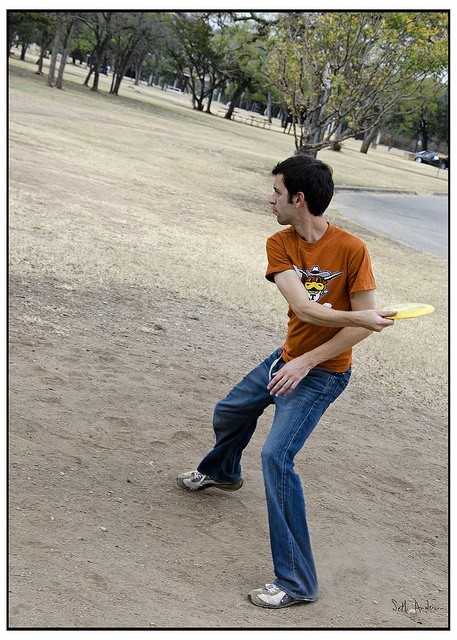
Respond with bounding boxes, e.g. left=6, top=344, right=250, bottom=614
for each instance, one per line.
left=248, top=583, right=310, bottom=611
left=177, top=470, right=245, bottom=493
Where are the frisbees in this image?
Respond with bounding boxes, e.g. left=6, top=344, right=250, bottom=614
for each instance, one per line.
left=381, top=303, right=435, bottom=320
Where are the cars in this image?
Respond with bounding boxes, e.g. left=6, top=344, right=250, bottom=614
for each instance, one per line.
left=412, top=151, right=448, bottom=169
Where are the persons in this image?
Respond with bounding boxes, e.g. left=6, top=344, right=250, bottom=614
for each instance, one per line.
left=177, top=155, right=397, bottom=609
